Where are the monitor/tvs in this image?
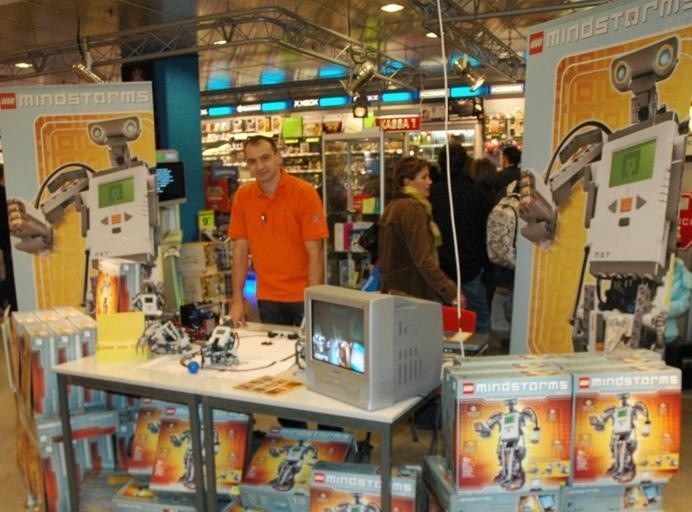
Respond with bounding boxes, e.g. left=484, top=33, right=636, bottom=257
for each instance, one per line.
left=303, top=284, right=443, bottom=412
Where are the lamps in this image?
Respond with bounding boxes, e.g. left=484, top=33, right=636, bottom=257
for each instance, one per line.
left=350, top=93, right=367, bottom=119
left=339, top=60, right=376, bottom=97
left=452, top=58, right=486, bottom=92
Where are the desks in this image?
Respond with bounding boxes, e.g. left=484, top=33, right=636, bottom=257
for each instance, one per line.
left=48, top=322, right=443, bottom=512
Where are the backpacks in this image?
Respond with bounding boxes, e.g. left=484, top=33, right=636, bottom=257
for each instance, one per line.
left=486, top=179, right=521, bottom=270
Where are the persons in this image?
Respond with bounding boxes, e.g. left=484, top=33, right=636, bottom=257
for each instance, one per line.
left=642, top=254, right=692, bottom=343
left=369, top=156, right=468, bottom=430
left=229, top=135, right=344, bottom=432
left=316, top=161, right=349, bottom=216
left=362, top=143, right=520, bottom=354
left=338, top=332, right=350, bottom=369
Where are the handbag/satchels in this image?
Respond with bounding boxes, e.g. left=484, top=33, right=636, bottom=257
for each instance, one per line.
left=358, top=222, right=379, bottom=255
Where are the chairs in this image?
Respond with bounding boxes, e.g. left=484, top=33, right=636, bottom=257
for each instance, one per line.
left=366, top=307, right=477, bottom=456
